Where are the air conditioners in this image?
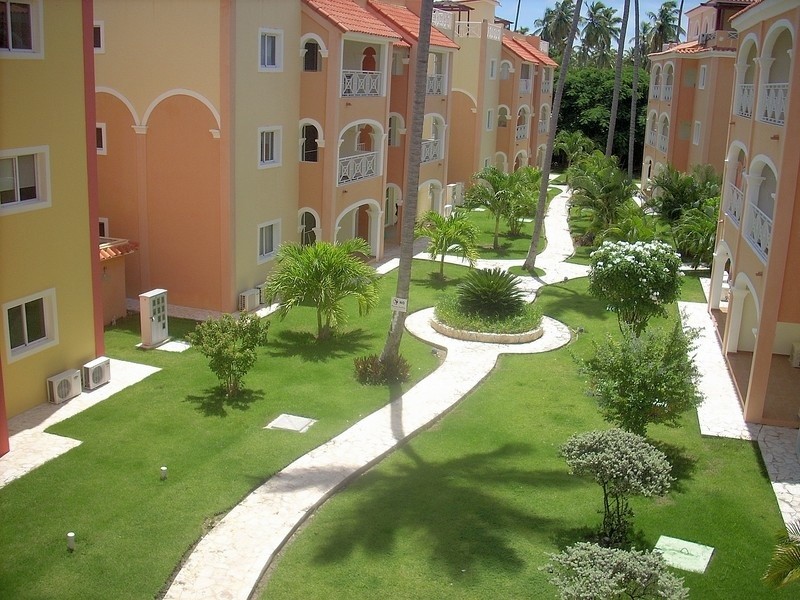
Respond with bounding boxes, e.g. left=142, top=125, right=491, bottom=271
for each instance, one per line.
left=46, top=368, right=82, bottom=404
left=238, top=289, right=260, bottom=312
left=82, top=356, right=111, bottom=389
left=256, top=284, right=266, bottom=304
